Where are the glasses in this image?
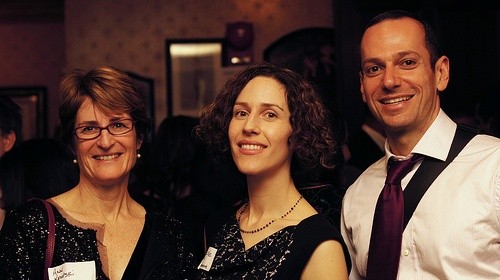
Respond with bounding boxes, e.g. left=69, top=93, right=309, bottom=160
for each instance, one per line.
left=72, top=118, right=136, bottom=140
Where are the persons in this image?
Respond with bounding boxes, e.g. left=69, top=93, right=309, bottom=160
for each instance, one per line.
left=0, top=67, right=196, bottom=280
left=0, top=88, right=24, bottom=232
left=340, top=11, right=500, bottom=280
left=194, top=63, right=353, bottom=280
left=150, top=115, right=210, bottom=266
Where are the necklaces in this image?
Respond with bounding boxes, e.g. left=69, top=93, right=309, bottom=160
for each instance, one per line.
left=237, top=195, right=303, bottom=234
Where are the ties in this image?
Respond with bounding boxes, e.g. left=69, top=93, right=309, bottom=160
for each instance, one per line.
left=365, top=154, right=425, bottom=280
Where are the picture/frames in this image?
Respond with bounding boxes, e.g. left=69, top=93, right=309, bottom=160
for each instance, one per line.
left=165, top=37, right=227, bottom=117
left=0, top=86, right=48, bottom=143
